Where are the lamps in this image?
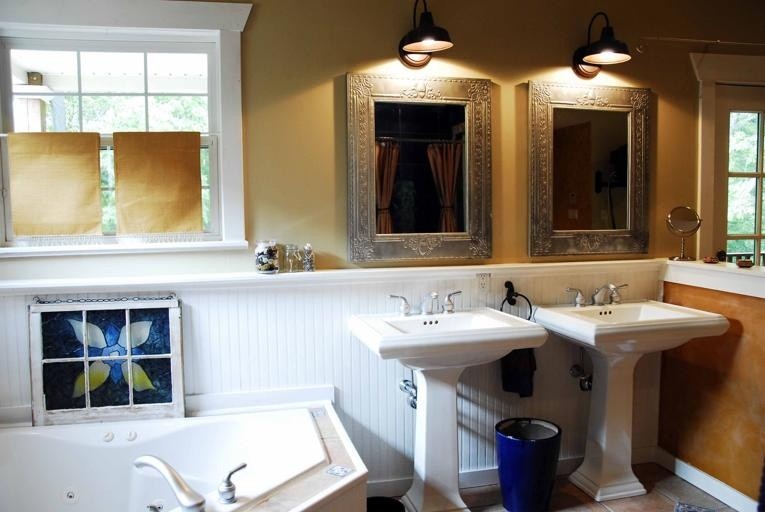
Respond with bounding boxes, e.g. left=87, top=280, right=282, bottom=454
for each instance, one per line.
left=395, top=0, right=454, bottom=68
left=571, top=9, right=632, bottom=78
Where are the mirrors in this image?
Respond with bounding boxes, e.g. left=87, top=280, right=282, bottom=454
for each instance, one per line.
left=666, top=204, right=703, bottom=261
left=347, top=71, right=492, bottom=260
left=524, top=78, right=650, bottom=253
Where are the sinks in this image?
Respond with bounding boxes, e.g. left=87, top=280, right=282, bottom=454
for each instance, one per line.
left=336, top=307, right=549, bottom=371
left=529, top=300, right=731, bottom=354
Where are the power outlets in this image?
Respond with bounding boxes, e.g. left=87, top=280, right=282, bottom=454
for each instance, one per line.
left=476, top=273, right=490, bottom=292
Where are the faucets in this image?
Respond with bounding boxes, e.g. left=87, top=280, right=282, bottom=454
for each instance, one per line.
left=422, top=292, right=439, bottom=316
left=592, top=283, right=619, bottom=305
left=134, top=455, right=205, bottom=512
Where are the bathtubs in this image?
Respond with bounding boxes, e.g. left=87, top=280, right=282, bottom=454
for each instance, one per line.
left=0, top=406, right=329, bottom=512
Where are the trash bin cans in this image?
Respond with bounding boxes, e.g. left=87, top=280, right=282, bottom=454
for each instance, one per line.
left=495, top=417, right=562, bottom=512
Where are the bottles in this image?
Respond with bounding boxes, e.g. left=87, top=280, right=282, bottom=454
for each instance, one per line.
left=282, top=244, right=302, bottom=272
left=254, top=239, right=280, bottom=272
left=301, top=244, right=316, bottom=272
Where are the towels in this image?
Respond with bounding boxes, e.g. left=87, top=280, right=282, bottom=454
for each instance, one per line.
left=501, top=348, right=537, bottom=397
left=5, top=128, right=106, bottom=240
left=113, top=131, right=204, bottom=235
